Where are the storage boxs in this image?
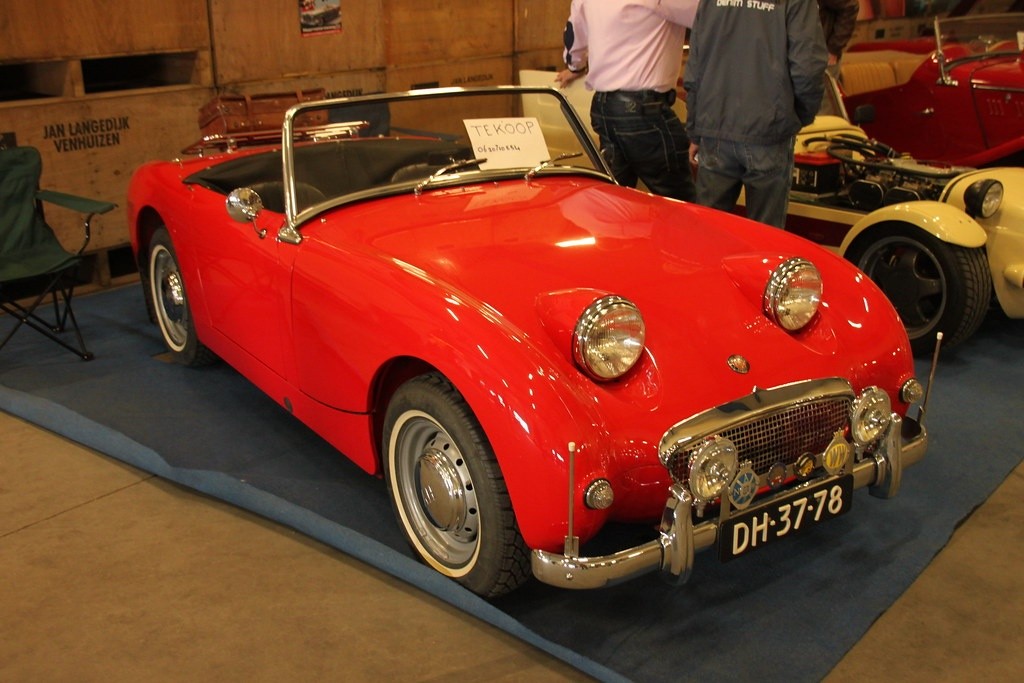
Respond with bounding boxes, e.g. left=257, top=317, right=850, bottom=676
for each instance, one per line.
left=793, top=150, right=841, bottom=194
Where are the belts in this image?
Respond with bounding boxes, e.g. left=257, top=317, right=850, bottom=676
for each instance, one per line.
left=594, top=91, right=666, bottom=102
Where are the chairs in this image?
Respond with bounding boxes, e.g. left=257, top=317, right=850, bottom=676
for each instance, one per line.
left=391, top=162, right=464, bottom=183
left=838, top=63, right=896, bottom=96
left=0, top=146, right=119, bottom=361
left=890, top=57, right=924, bottom=85
left=240, top=182, right=326, bottom=214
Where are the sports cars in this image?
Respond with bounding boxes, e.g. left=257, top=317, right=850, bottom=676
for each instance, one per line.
left=127, top=82, right=951, bottom=600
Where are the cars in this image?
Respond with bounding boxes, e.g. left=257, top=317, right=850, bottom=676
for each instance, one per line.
left=516, top=8, right=1024, bottom=356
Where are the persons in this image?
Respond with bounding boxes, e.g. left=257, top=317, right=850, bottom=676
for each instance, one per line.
left=554, top=1, right=859, bottom=231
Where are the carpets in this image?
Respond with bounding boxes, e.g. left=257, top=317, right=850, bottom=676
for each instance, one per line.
left=0, top=281, right=1024, bottom=683
